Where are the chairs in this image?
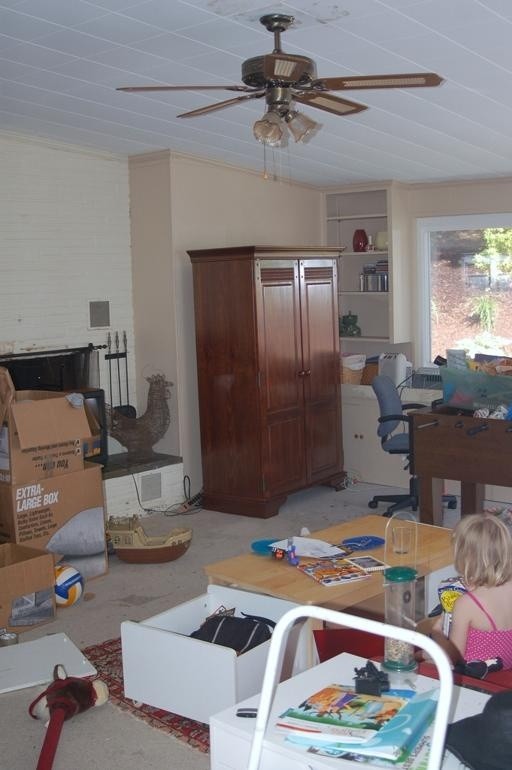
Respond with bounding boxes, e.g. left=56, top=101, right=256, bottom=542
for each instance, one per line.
left=370, top=376, right=457, bottom=516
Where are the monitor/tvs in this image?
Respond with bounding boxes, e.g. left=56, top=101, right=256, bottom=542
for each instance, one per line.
left=64, top=386, right=109, bottom=471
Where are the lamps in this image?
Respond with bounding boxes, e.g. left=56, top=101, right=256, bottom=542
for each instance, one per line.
left=254, top=103, right=322, bottom=148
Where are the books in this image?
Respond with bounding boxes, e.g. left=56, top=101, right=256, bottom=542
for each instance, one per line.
left=273, top=678, right=439, bottom=770
left=295, top=556, right=371, bottom=587
left=1, top=630, right=97, bottom=696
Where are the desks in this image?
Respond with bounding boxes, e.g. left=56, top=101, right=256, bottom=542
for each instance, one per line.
left=407, top=398, right=512, bottom=525
left=202, top=514, right=454, bottom=629
left=207, top=651, right=493, bottom=770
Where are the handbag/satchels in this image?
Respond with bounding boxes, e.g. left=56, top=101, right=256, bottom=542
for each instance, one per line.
left=187, top=613, right=279, bottom=657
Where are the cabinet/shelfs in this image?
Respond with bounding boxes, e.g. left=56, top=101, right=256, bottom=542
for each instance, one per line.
left=186, top=245, right=348, bottom=519
left=121, top=584, right=310, bottom=725
left=319, top=180, right=415, bottom=344
left=342, top=384, right=408, bottom=491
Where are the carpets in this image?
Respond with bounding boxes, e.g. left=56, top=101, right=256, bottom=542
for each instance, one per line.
left=81, top=608, right=422, bottom=752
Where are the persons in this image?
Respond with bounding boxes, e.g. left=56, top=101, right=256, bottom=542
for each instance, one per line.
left=430, top=510, right=511, bottom=673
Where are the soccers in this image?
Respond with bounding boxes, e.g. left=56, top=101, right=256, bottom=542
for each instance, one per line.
left=55, top=565, right=85, bottom=605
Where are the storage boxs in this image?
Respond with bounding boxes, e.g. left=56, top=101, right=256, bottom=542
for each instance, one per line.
left=0, top=368, right=109, bottom=635
left=439, top=364, right=512, bottom=411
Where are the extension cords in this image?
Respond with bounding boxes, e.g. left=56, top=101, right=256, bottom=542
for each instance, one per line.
left=177, top=491, right=203, bottom=515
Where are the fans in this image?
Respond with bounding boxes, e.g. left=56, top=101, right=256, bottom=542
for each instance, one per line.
left=115, top=13, right=445, bottom=119
